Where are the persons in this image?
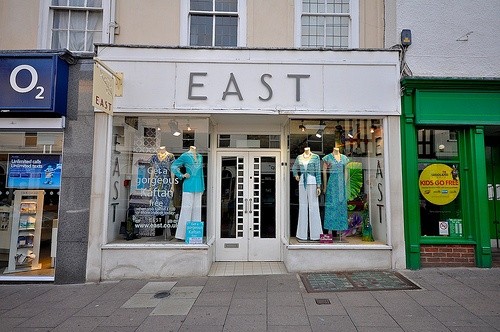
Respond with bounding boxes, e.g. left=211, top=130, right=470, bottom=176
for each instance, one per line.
left=148, top=146, right=176, bottom=238
left=292, top=146, right=324, bottom=240
left=321, top=148, right=349, bottom=237
left=170, top=145, right=205, bottom=243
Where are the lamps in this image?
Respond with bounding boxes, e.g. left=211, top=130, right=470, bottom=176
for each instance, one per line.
left=315, top=120, right=326, bottom=138
left=346, top=124, right=360, bottom=139
left=169, top=119, right=181, bottom=136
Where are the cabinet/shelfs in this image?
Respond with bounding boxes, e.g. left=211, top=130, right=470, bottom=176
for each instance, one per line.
left=15, top=195, right=38, bottom=269
